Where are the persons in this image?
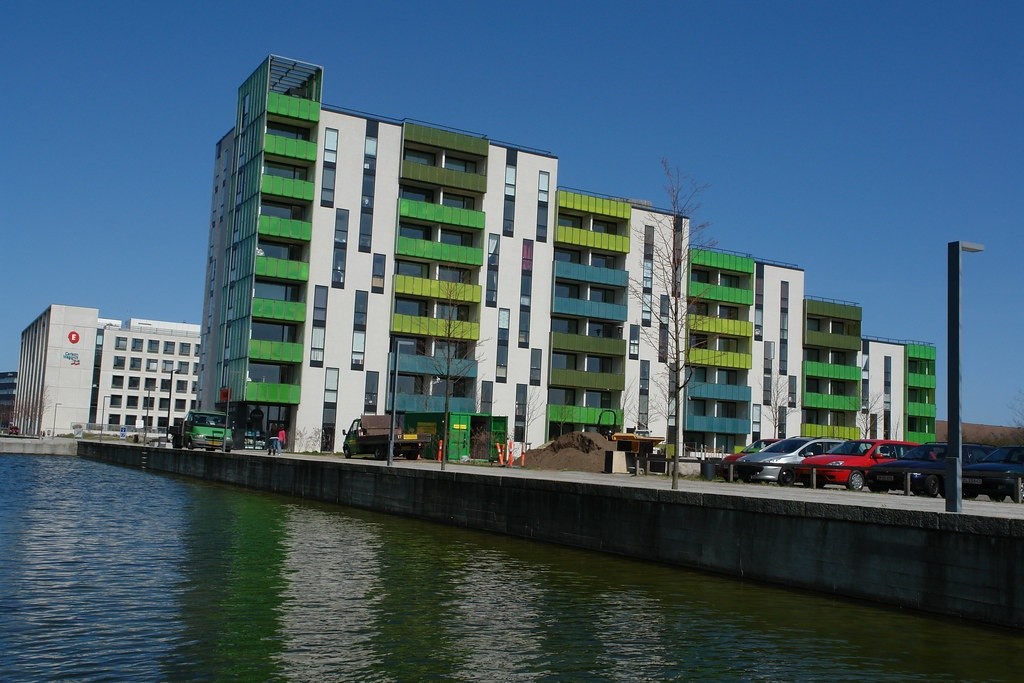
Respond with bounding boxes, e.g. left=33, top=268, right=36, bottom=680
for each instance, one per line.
left=268, top=423, right=286, bottom=455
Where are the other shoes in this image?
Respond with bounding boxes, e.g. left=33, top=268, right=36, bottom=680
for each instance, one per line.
left=278, top=452, right=280, bottom=454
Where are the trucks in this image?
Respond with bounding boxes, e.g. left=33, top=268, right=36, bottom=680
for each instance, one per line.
left=342, top=413, right=431, bottom=460
left=169, top=409, right=233, bottom=452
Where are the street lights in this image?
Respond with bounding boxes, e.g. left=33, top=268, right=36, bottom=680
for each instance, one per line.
left=52, top=403, right=61, bottom=439
left=99, top=395, right=110, bottom=441
left=223, top=371, right=242, bottom=451
left=166, top=368, right=181, bottom=442
left=944, top=239, right=984, bottom=513
left=387, top=340, right=414, bottom=468
left=144, top=386, right=159, bottom=447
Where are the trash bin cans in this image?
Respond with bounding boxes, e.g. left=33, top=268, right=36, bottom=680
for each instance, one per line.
left=133, top=435, right=139, bottom=443
left=158, top=437, right=167, bottom=448
left=701, top=460, right=715, bottom=479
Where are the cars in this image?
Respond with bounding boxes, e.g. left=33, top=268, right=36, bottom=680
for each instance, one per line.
left=962, top=445, right=1024, bottom=503
left=720, top=439, right=782, bottom=482
left=798, top=439, right=936, bottom=492
left=867, top=441, right=999, bottom=499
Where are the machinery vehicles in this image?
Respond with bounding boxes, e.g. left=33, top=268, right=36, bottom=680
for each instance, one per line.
left=597, top=410, right=664, bottom=456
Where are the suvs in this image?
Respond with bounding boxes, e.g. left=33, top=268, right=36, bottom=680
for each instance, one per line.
left=9, top=426, right=18, bottom=435
left=735, top=436, right=864, bottom=488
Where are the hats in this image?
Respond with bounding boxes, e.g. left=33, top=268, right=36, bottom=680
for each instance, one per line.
left=279, top=425, right=284, bottom=428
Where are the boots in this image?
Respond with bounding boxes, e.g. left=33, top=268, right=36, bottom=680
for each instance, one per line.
left=268, top=450, right=271, bottom=455
left=273, top=450, right=275, bottom=455
